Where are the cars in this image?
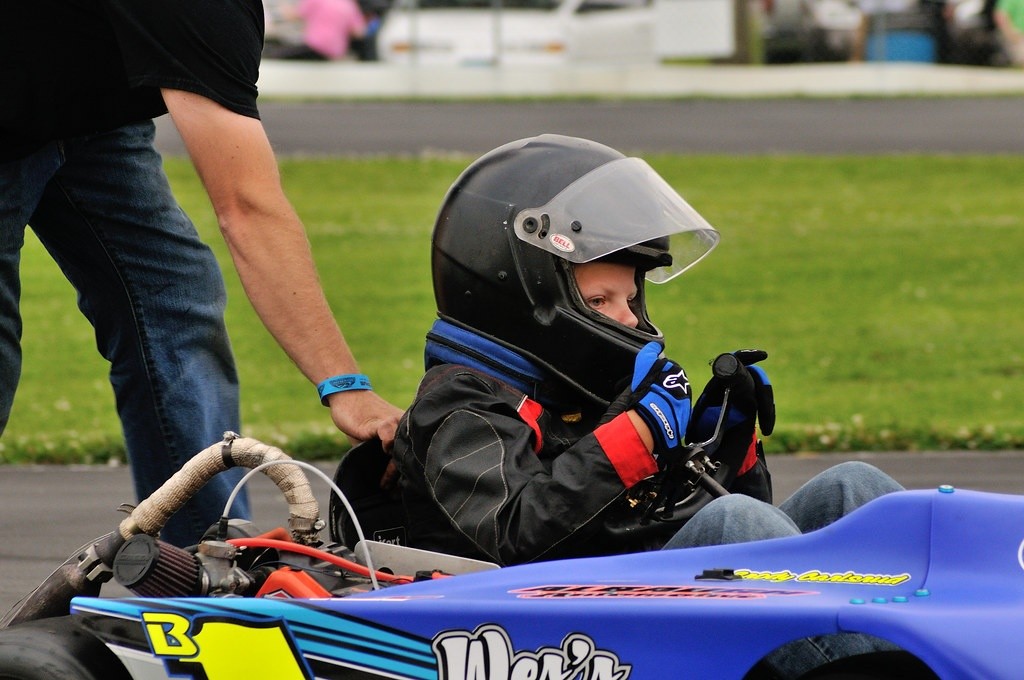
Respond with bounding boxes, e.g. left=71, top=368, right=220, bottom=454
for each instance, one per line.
left=377, top=0, right=734, bottom=76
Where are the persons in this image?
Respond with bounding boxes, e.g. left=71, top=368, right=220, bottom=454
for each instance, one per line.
left=359, top=133, right=907, bottom=567
left=0, top=0, right=407, bottom=600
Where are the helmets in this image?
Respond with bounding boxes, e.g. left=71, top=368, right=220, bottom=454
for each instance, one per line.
left=431, top=133, right=719, bottom=413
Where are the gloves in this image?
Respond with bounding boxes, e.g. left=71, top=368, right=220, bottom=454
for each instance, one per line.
left=630, top=340, right=693, bottom=459
left=684, top=351, right=776, bottom=440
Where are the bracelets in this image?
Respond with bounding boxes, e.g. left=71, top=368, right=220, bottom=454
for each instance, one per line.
left=316, top=372, right=372, bottom=407
